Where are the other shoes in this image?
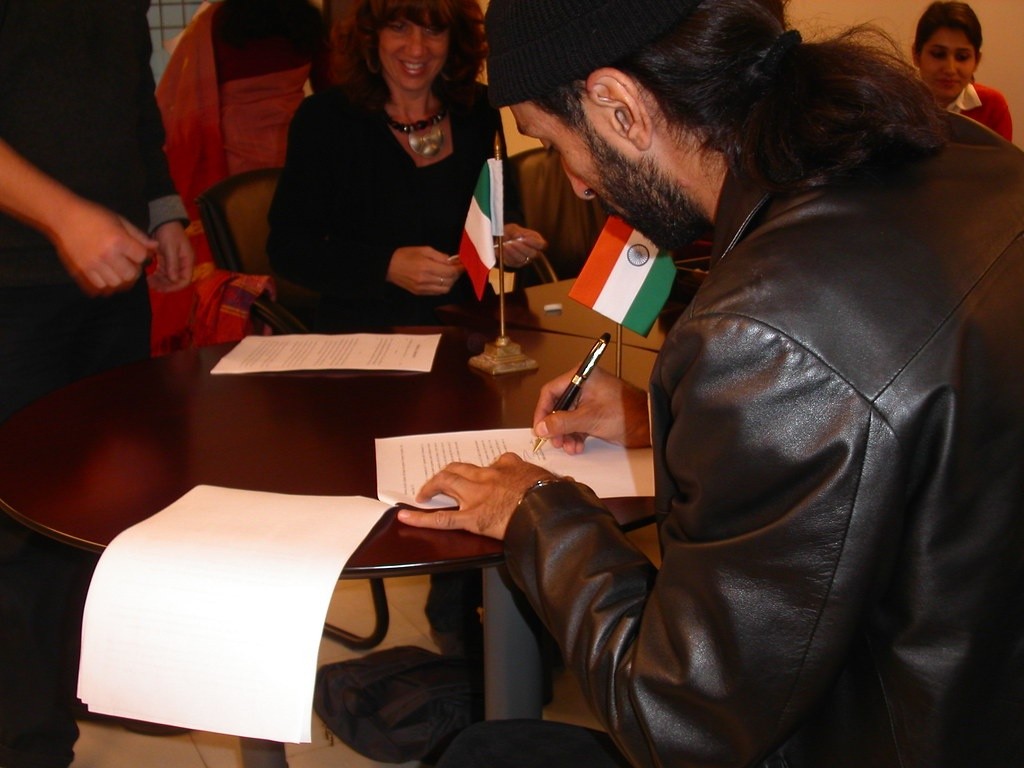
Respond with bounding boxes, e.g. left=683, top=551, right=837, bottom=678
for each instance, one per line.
left=431, top=623, right=484, bottom=668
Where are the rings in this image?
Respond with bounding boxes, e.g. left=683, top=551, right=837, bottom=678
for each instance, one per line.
left=440, top=277, right=443, bottom=286
left=523, top=257, right=529, bottom=264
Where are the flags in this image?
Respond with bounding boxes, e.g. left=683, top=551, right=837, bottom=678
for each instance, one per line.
left=568, top=216, right=677, bottom=338
left=457, top=158, right=504, bottom=301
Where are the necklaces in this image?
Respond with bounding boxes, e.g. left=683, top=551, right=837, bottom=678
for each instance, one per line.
left=385, top=110, right=446, bottom=159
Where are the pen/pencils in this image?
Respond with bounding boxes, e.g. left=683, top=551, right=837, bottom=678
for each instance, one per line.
left=446, top=237, right=525, bottom=262
left=532, top=332, right=612, bottom=456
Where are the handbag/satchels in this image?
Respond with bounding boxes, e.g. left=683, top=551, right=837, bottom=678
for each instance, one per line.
left=312, top=646, right=484, bottom=764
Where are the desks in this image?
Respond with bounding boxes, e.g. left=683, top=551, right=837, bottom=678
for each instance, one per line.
left=0, top=325, right=663, bottom=768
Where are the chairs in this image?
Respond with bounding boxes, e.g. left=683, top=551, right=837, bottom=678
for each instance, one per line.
left=200, top=164, right=308, bottom=330
left=512, top=146, right=615, bottom=267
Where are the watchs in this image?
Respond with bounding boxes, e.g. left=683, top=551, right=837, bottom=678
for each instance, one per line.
left=517, top=478, right=559, bottom=505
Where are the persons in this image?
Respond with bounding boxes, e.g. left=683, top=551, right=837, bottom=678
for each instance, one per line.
left=911, top=0, right=1013, bottom=143
left=398, top=0, right=1024, bottom=768
left=263, top=0, right=547, bottom=654
left=0, top=0, right=196, bottom=768
